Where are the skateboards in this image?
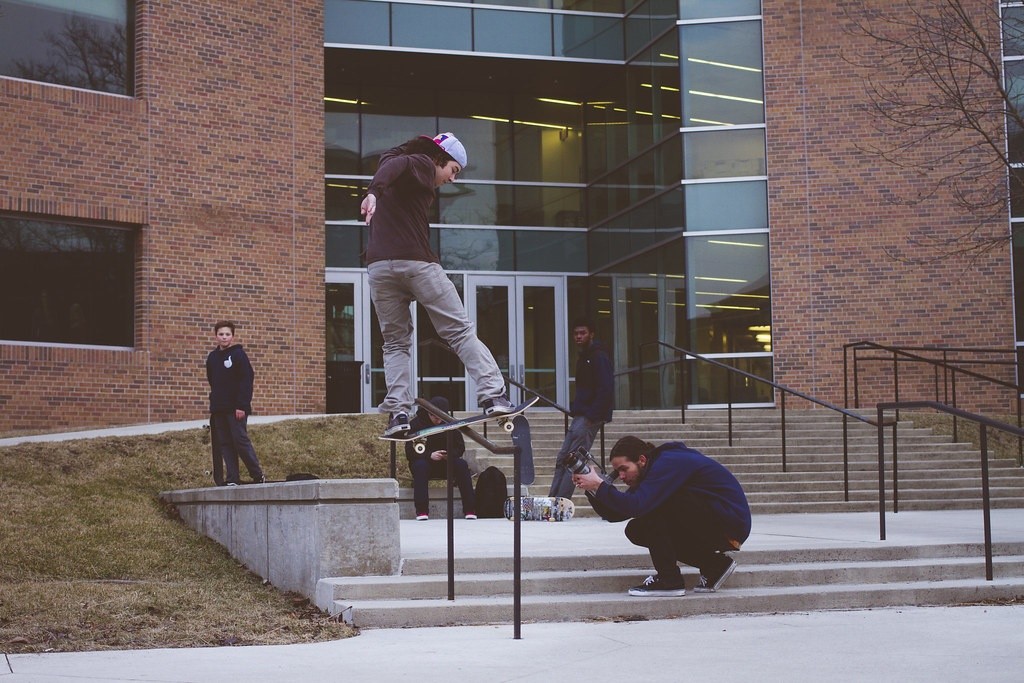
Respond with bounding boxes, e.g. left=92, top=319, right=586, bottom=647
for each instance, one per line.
left=510, top=415, right=535, bottom=485
left=504, top=495, right=575, bottom=522
left=376, top=394, right=538, bottom=455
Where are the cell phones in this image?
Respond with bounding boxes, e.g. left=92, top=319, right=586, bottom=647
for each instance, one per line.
left=441, top=452, right=447, bottom=455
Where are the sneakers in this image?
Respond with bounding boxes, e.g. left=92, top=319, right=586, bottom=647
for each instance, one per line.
left=227, top=482, right=240, bottom=485
left=415, top=515, right=428, bottom=520
left=483, top=397, right=516, bottom=414
left=383, top=412, right=411, bottom=439
left=254, top=476, right=265, bottom=483
left=627, top=569, right=688, bottom=598
left=465, top=513, right=476, bottom=519
left=693, top=556, right=738, bottom=593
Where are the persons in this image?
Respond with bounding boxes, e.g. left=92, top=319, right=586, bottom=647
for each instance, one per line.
left=205, top=320, right=267, bottom=486
left=571, top=435, right=754, bottom=597
left=357, top=130, right=517, bottom=437
left=404, top=396, right=480, bottom=520
left=547, top=319, right=615, bottom=502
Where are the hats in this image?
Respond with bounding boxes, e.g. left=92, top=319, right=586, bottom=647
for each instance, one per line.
left=419, top=129, right=468, bottom=169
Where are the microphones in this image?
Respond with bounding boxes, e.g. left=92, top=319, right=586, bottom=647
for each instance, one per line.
left=578, top=446, right=593, bottom=461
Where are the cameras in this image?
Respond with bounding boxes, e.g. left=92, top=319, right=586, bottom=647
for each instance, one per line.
left=564, top=450, right=619, bottom=498
